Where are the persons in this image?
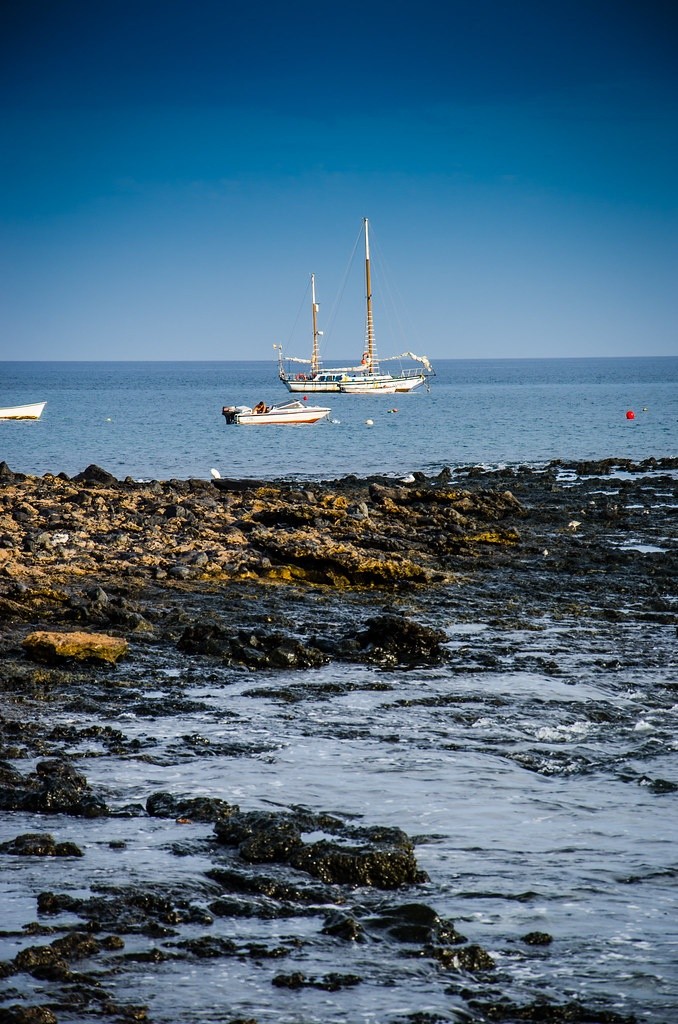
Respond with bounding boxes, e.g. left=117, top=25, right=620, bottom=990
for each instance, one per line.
left=254, top=401, right=267, bottom=414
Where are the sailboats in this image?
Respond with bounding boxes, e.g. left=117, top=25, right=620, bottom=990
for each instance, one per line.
left=311, top=217, right=436, bottom=394
left=273, top=272, right=364, bottom=392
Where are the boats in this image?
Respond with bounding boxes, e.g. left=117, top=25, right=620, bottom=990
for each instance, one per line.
left=0, top=400, right=47, bottom=422
left=222, top=397, right=331, bottom=425
